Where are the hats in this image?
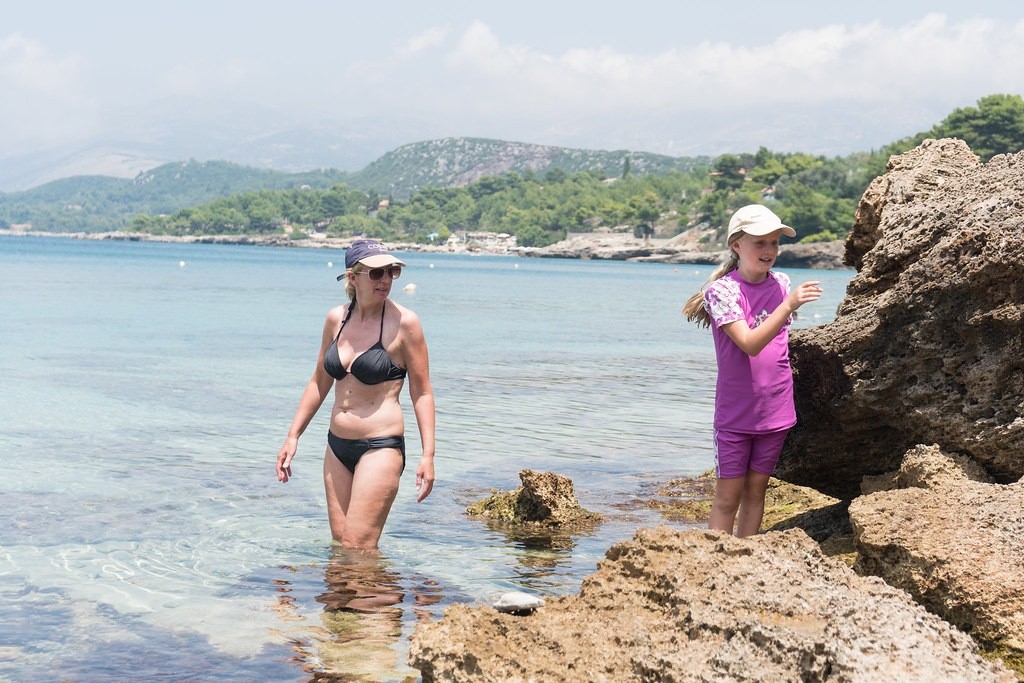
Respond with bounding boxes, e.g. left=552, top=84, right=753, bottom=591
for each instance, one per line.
left=337, top=240, right=406, bottom=282
left=727, top=205, right=796, bottom=246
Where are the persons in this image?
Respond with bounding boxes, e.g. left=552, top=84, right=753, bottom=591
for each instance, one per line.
left=275, top=237, right=438, bottom=548
left=268, top=550, right=440, bottom=681
left=682, top=205, right=826, bottom=536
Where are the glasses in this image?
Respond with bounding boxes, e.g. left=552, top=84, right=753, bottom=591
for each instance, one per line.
left=354, top=265, right=401, bottom=281
